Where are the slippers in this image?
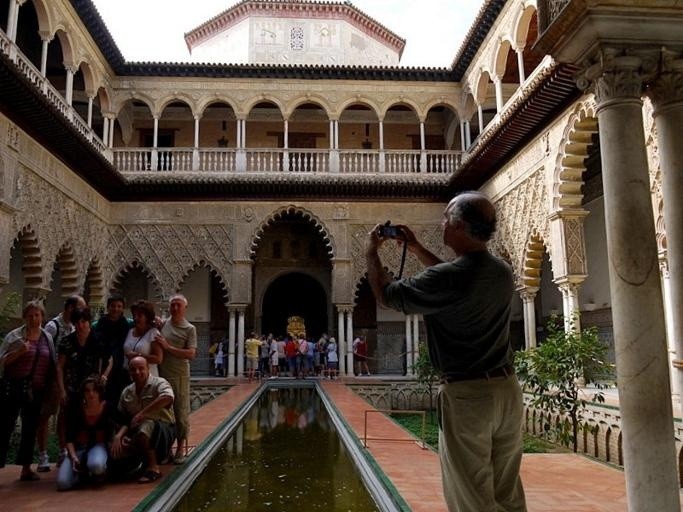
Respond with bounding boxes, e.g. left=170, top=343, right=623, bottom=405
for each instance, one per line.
left=19, top=472, right=40, bottom=479
left=138, top=471, right=162, bottom=484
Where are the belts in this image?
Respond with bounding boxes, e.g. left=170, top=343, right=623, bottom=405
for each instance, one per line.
left=440, top=367, right=514, bottom=384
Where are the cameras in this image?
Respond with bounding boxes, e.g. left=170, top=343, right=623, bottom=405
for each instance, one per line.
left=379, top=225, right=398, bottom=237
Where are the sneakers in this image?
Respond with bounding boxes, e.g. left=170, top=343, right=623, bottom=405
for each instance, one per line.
left=37, top=454, right=50, bottom=472
left=173, top=454, right=184, bottom=464
left=56, top=451, right=68, bottom=467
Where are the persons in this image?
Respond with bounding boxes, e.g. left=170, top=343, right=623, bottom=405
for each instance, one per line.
left=365, top=190, right=526, bottom=512
left=0, top=293, right=197, bottom=491
left=256, top=387, right=319, bottom=435
left=212, top=332, right=372, bottom=383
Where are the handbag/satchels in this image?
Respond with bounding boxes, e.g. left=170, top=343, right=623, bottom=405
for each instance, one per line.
left=1, top=376, right=33, bottom=405
